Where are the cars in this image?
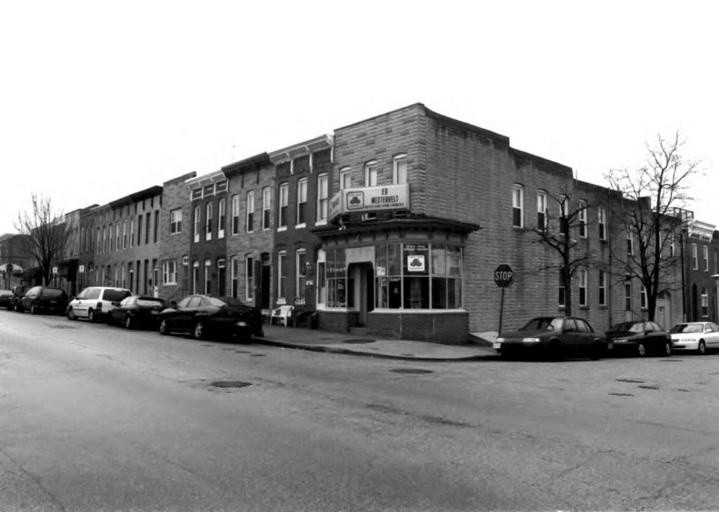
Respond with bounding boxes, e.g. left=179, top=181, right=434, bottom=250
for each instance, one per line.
left=1, top=284, right=263, bottom=340
left=493, top=316, right=719, bottom=362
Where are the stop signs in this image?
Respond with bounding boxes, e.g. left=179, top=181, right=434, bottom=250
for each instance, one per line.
left=494, top=264, right=514, bottom=288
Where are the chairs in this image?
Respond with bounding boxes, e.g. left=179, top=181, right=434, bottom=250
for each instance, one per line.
left=270, top=304, right=295, bottom=328
